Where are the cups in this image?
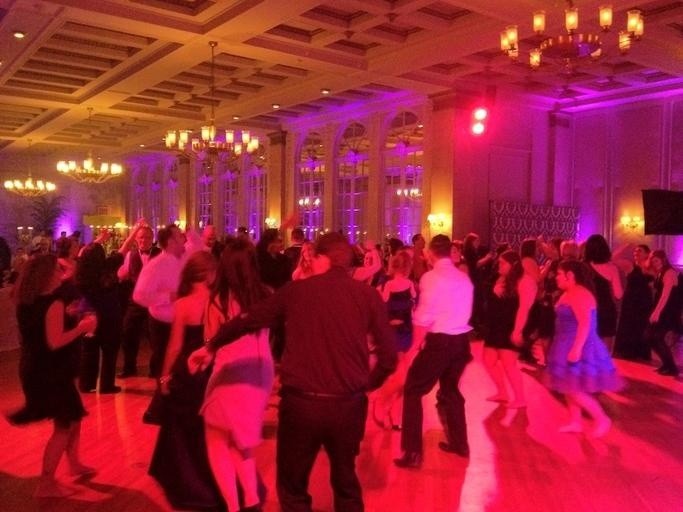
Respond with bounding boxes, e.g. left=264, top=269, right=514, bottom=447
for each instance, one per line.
left=83, top=311, right=97, bottom=338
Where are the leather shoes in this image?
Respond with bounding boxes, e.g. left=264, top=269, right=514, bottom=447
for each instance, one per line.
left=438, top=439, right=470, bottom=458
left=393, top=455, right=422, bottom=469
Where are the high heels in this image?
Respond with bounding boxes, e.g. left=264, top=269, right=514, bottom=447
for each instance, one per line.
left=372, top=396, right=386, bottom=429
left=388, top=406, right=402, bottom=431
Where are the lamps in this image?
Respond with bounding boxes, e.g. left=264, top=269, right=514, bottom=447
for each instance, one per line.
left=4, top=139, right=57, bottom=197
left=427, top=213, right=449, bottom=229
left=57, top=108, right=122, bottom=184
left=469, top=105, right=490, bottom=137
left=621, top=216, right=640, bottom=230
left=500, top=0, right=644, bottom=75
left=163, top=40, right=269, bottom=169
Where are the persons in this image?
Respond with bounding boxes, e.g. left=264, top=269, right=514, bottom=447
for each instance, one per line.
left=410, top=234, right=430, bottom=279
left=363, top=252, right=381, bottom=286
left=57, top=231, right=70, bottom=256
left=202, top=239, right=272, bottom=512
left=282, top=228, right=305, bottom=258
left=131, top=224, right=185, bottom=424
left=144, top=250, right=227, bottom=510
left=292, top=242, right=314, bottom=280
left=258, top=228, right=292, bottom=288
left=375, top=253, right=416, bottom=353
left=116, top=226, right=162, bottom=379
left=477, top=251, right=537, bottom=409
left=612, top=241, right=650, bottom=358
left=187, top=231, right=398, bottom=512
left=68, top=231, right=80, bottom=256
left=451, top=241, right=468, bottom=273
left=521, top=240, right=553, bottom=364
left=464, top=231, right=492, bottom=281
left=560, top=240, right=593, bottom=288
left=32, top=229, right=46, bottom=252
left=581, top=233, right=623, bottom=357
left=10, top=253, right=96, bottom=501
left=78, top=219, right=144, bottom=395
left=648, top=250, right=683, bottom=375
left=394, top=233, right=473, bottom=470
left=544, top=260, right=618, bottom=436
